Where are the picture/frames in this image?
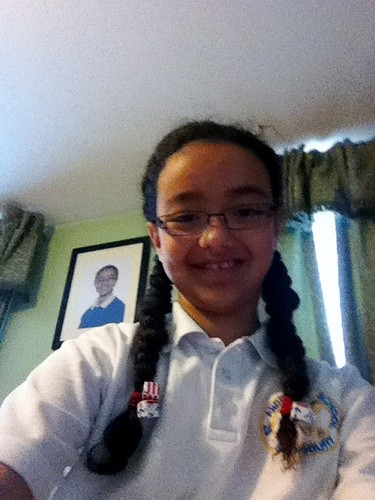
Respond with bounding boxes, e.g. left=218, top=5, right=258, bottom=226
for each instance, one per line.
left=51, top=234, right=151, bottom=351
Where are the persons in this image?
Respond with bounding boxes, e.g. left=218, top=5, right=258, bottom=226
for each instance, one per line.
left=77, top=265, right=126, bottom=330
left=0, top=121, right=375, bottom=500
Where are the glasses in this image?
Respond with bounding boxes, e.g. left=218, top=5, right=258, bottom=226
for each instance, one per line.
left=153, top=204, right=276, bottom=236
